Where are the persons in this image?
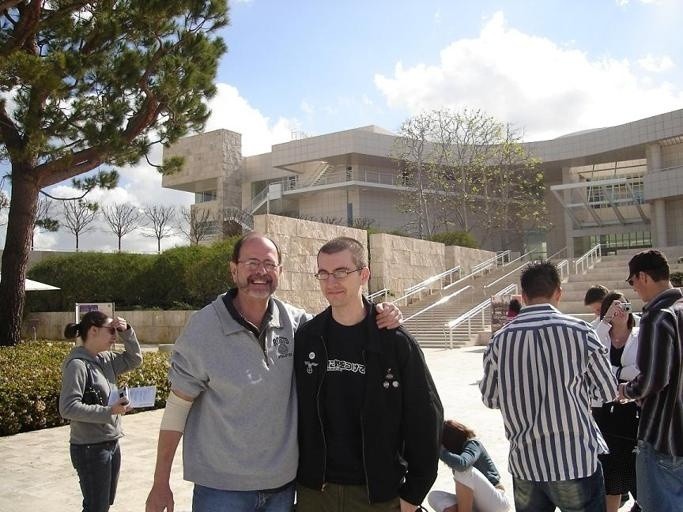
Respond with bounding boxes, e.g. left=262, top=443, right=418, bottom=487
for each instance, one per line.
left=59, top=311, right=142, bottom=512
left=428, top=420, right=510, bottom=512
left=146, top=231, right=405, bottom=512
left=291, top=238, right=444, bottom=512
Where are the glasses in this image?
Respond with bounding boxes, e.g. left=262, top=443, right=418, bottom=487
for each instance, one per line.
left=237, top=259, right=279, bottom=273
left=96, top=325, right=116, bottom=335
left=314, top=268, right=363, bottom=280
left=628, top=279, right=634, bottom=286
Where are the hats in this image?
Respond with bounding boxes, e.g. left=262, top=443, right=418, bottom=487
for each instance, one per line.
left=626, top=247, right=668, bottom=282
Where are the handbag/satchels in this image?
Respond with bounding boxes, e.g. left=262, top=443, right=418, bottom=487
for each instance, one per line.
left=82, top=387, right=107, bottom=406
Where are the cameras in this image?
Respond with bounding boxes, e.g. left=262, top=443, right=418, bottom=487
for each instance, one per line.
left=619, top=303, right=632, bottom=313
left=118, top=386, right=129, bottom=406
